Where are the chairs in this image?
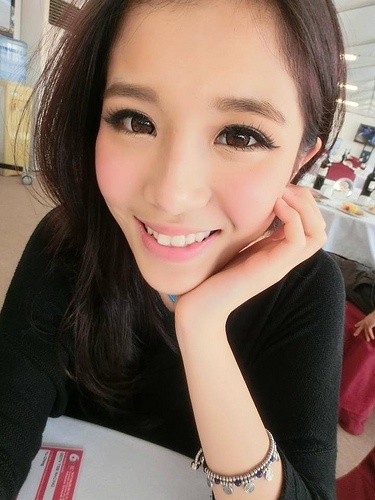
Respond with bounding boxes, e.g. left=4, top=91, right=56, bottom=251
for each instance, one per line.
left=325, top=162, right=356, bottom=182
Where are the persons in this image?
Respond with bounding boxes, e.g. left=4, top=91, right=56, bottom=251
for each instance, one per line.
left=0, top=0, right=345, bottom=500
left=352, top=307, right=375, bottom=342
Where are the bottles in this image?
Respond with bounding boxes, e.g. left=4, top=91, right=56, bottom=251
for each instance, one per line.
left=314, top=153, right=330, bottom=190
left=361, top=167, right=375, bottom=196
left=0, top=34, right=27, bottom=83
left=341, top=147, right=351, bottom=163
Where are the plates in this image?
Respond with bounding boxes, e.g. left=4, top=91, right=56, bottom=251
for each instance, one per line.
left=353, top=200, right=375, bottom=215
left=336, top=207, right=360, bottom=216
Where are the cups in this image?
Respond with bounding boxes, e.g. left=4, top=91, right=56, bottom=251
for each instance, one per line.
left=358, top=195, right=371, bottom=204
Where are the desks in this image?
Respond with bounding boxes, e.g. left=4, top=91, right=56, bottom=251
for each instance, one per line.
left=297, top=173, right=375, bottom=270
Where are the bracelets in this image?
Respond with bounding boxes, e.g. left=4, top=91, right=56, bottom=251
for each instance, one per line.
left=188, top=429, right=281, bottom=494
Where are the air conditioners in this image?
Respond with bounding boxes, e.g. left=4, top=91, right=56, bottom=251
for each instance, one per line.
left=22, top=0, right=81, bottom=171
